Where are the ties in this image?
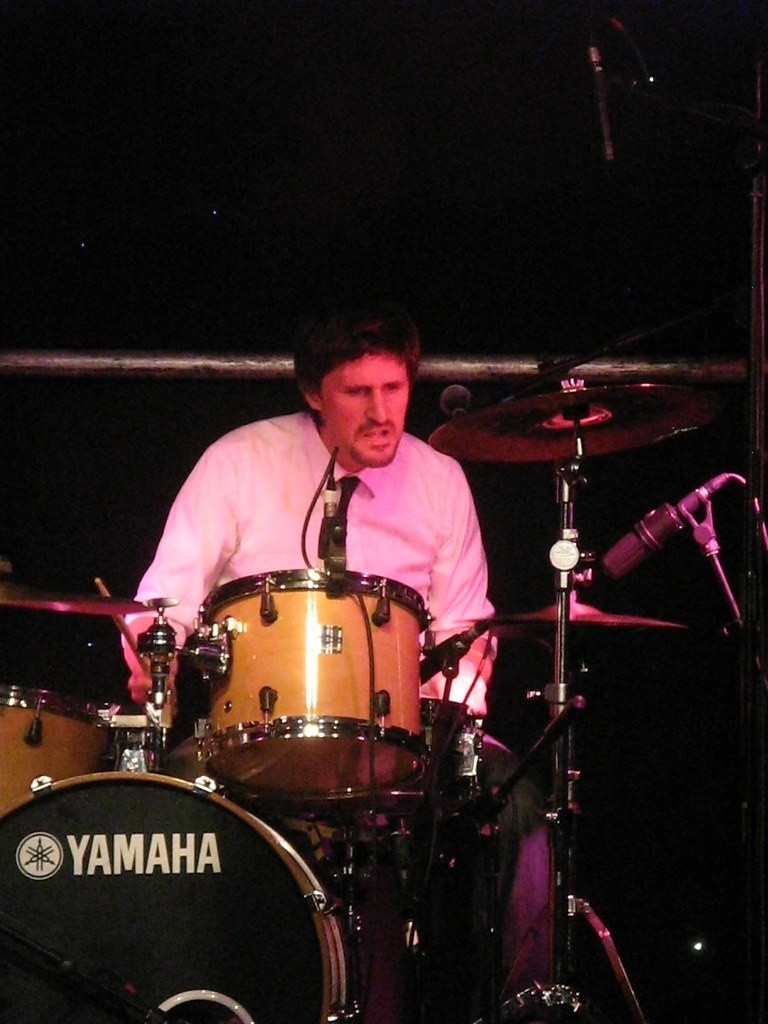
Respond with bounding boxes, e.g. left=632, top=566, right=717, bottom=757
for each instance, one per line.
left=338, top=474, right=360, bottom=523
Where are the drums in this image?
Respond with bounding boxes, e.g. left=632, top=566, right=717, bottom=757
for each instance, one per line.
left=1, top=771, right=348, bottom=1024
left=418, top=696, right=482, bottom=776
left=0, top=684, right=111, bottom=812
left=191, top=569, right=435, bottom=801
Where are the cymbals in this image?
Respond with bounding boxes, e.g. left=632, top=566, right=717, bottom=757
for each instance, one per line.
left=0, top=588, right=165, bottom=615
left=426, top=380, right=746, bottom=464
left=455, top=602, right=687, bottom=631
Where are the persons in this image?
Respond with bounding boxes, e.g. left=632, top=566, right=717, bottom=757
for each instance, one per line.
left=118, top=311, right=500, bottom=1022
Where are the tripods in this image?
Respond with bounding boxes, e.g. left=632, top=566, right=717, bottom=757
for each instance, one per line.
left=493, top=411, right=649, bottom=1024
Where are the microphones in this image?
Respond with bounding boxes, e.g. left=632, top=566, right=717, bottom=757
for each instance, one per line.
left=441, top=384, right=471, bottom=418
left=317, top=476, right=339, bottom=560
left=421, top=621, right=491, bottom=684
left=599, top=474, right=728, bottom=581
